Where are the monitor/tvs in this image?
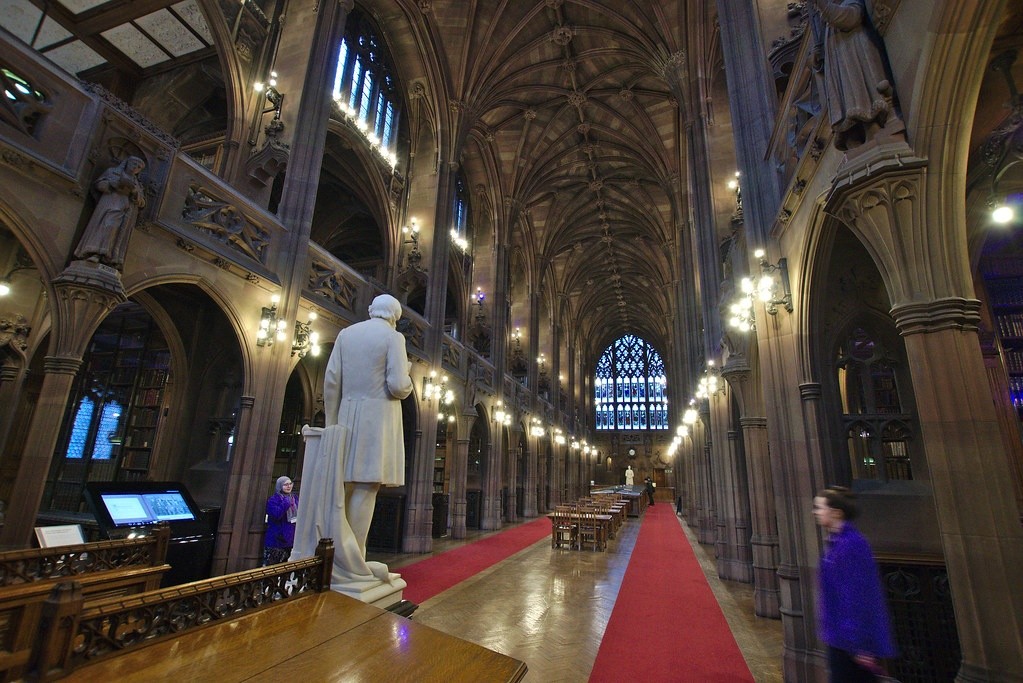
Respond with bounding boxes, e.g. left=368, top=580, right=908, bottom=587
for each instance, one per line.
left=102, top=493, right=197, bottom=528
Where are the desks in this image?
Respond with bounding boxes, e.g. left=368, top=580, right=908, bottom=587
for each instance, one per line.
left=545, top=498, right=630, bottom=551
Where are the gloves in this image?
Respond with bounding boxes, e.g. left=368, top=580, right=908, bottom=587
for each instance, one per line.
left=285, top=497, right=291, bottom=505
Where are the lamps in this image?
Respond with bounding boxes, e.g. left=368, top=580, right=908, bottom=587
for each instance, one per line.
left=253, top=70, right=285, bottom=120
left=754, top=248, right=793, bottom=316
left=256, top=293, right=323, bottom=359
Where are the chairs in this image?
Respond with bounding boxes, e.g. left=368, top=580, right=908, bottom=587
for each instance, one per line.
left=551, top=491, right=619, bottom=553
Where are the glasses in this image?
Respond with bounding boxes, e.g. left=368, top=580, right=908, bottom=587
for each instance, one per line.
left=284, top=483, right=292, bottom=486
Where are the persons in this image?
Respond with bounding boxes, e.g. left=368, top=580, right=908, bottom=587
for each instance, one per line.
left=262, top=477, right=299, bottom=591
left=75, top=155, right=146, bottom=263
left=805, top=0, right=886, bottom=129
left=645, top=477, right=654, bottom=505
left=813, top=485, right=893, bottom=683
left=324, top=294, right=413, bottom=578
left=624, top=465, right=634, bottom=485
